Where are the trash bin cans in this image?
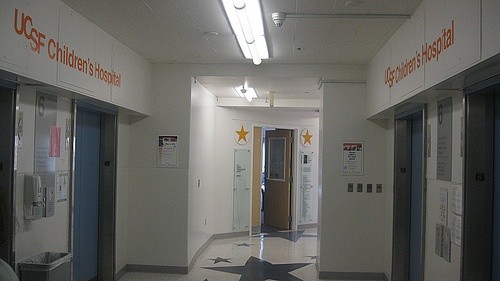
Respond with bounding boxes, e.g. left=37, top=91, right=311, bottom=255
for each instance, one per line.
left=17, top=250, right=73, bottom=281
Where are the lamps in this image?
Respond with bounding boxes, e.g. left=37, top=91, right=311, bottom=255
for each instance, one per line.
left=221, top=0, right=270, bottom=65
left=233, top=82, right=258, bottom=103
